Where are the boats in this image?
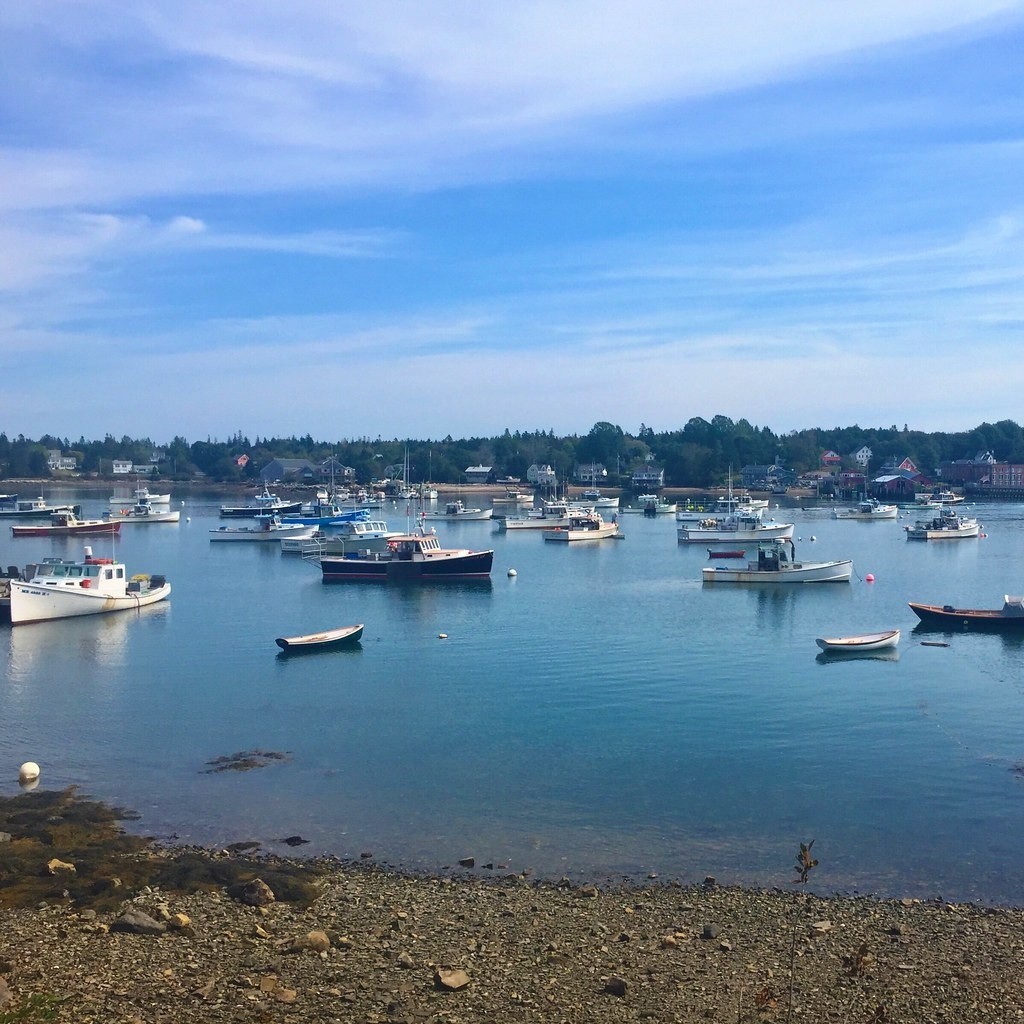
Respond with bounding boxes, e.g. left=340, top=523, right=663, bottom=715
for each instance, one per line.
left=492, top=461, right=625, bottom=541
left=815, top=627, right=902, bottom=652
left=103, top=486, right=181, bottom=523
left=703, top=539, right=854, bottom=583
left=623, top=494, right=676, bottom=513
left=276, top=623, right=365, bottom=651
left=908, top=594, right=1024, bottom=628
left=816, top=646, right=901, bottom=665
left=415, top=475, right=493, bottom=522
left=831, top=477, right=897, bottom=519
left=108, top=488, right=171, bottom=503
left=898, top=501, right=943, bottom=510
left=928, top=487, right=965, bottom=504
left=0, top=523, right=172, bottom=626
left=675, top=463, right=795, bottom=544
left=0, top=494, right=82, bottom=518
left=208, top=440, right=494, bottom=579
left=9, top=509, right=122, bottom=535
left=902, top=506, right=980, bottom=541
